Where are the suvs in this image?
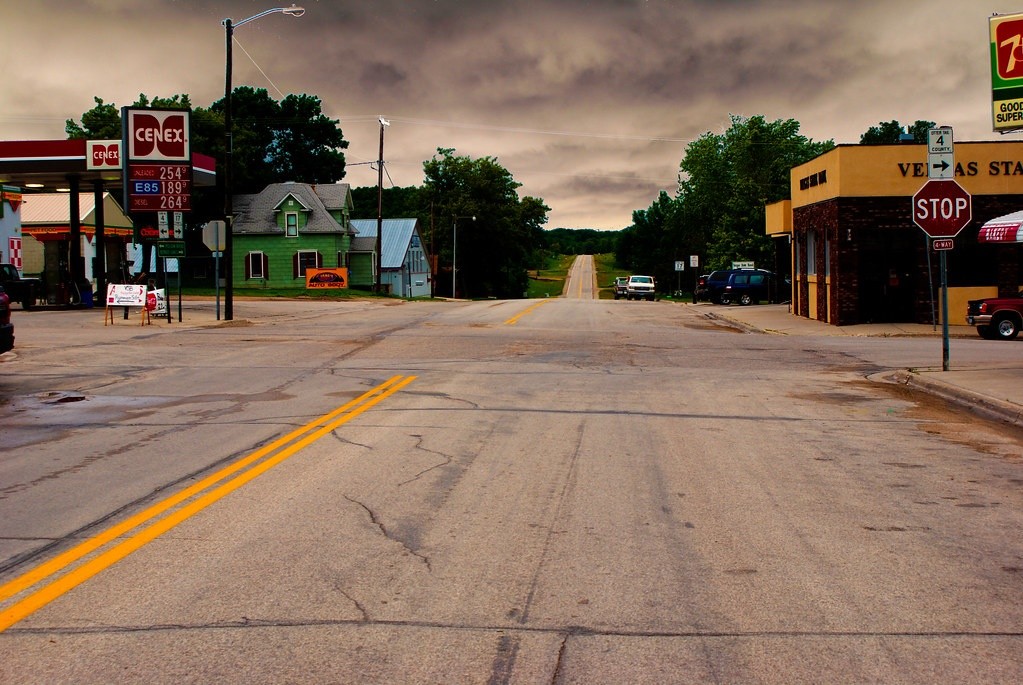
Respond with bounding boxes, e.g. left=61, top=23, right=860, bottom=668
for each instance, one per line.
left=612, top=276, right=635, bottom=300
left=624, top=274, right=658, bottom=301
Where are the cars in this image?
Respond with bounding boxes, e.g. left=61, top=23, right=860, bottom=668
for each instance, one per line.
left=966, top=274, right=1023, bottom=341
left=0, top=292, right=16, bottom=354
left=692, top=267, right=796, bottom=305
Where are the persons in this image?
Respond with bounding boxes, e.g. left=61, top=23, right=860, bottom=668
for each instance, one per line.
left=148, top=279, right=156, bottom=291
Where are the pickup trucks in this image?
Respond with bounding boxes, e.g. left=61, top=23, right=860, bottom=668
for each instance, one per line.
left=0, top=261, right=42, bottom=312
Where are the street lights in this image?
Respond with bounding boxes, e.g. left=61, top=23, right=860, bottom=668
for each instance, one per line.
left=222, top=6, right=306, bottom=320
left=453, top=213, right=477, bottom=297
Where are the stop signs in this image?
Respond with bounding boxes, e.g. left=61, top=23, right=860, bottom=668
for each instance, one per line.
left=912, top=178, right=972, bottom=239
